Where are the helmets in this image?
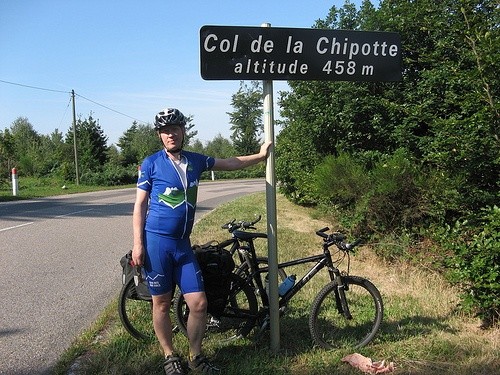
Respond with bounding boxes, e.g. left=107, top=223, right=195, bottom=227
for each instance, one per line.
left=154, top=107, right=186, bottom=129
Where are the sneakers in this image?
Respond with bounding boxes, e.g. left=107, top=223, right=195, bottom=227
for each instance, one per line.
left=186, top=352, right=227, bottom=375
left=163, top=350, right=186, bottom=375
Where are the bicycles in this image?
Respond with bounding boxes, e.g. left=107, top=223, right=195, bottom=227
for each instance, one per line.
left=118, top=215, right=288, bottom=342
left=176, top=225, right=384, bottom=351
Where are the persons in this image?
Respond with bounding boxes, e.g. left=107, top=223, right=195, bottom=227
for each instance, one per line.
left=131, top=107, right=273, bottom=374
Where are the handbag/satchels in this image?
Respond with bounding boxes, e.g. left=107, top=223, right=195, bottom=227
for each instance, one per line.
left=120, top=249, right=154, bottom=301
left=192, top=240, right=235, bottom=316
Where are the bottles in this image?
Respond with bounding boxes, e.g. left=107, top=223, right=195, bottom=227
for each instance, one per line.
left=265, top=273, right=271, bottom=304
left=278, top=274, right=296, bottom=297
